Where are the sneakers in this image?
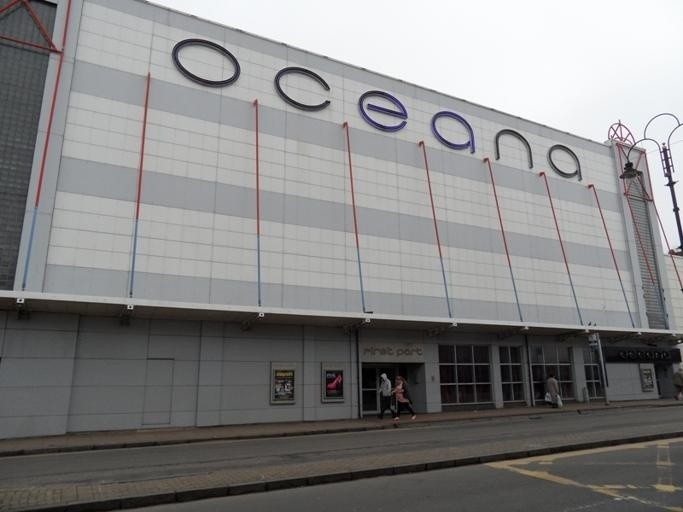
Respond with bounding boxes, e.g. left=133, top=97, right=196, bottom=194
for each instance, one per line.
left=674, top=395, right=680, bottom=400
left=376, top=414, right=416, bottom=421
left=551, top=404, right=558, bottom=408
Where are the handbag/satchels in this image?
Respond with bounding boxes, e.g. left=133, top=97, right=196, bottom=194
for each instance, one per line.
left=545, top=393, right=552, bottom=402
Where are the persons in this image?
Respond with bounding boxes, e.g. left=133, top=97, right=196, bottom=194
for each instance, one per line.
left=546, top=372, right=559, bottom=409
left=391, top=375, right=417, bottom=421
left=671, top=367, right=683, bottom=401
left=374, top=373, right=398, bottom=419
